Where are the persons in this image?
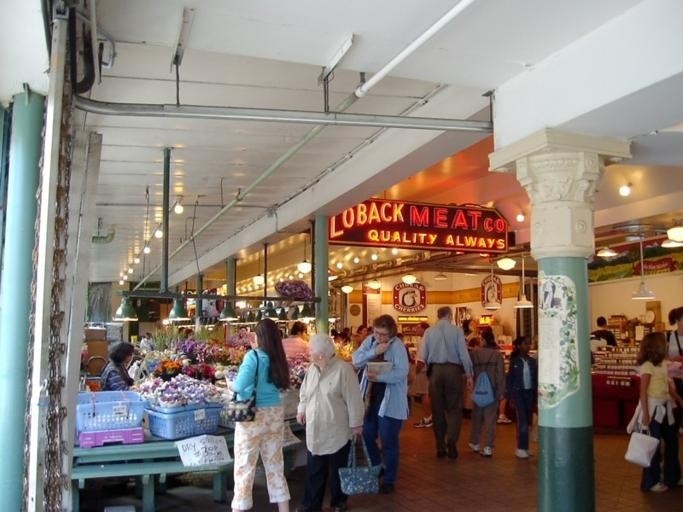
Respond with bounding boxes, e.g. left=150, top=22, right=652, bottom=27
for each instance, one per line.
left=506, top=336, right=538, bottom=458
left=291, top=322, right=307, bottom=336
left=145, top=324, right=253, bottom=339
left=469, top=332, right=505, bottom=457
left=225, top=318, right=290, bottom=512
left=100, top=342, right=131, bottom=391
left=397, top=333, right=411, bottom=418
left=590, top=316, right=618, bottom=347
left=627, top=331, right=682, bottom=494
left=412, top=322, right=433, bottom=428
left=662, top=306, right=683, bottom=434
left=296, top=333, right=364, bottom=511
left=330, top=324, right=373, bottom=347
left=350, top=314, right=409, bottom=494
left=414, top=306, right=473, bottom=458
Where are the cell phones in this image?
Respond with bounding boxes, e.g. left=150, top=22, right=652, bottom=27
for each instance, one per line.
left=388, top=339, right=394, bottom=344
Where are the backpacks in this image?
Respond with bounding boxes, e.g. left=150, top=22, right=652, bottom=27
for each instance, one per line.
left=471, top=371, right=493, bottom=407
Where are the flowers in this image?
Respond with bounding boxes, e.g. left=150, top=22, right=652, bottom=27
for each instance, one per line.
left=274, top=278, right=312, bottom=298
left=133, top=326, right=354, bottom=410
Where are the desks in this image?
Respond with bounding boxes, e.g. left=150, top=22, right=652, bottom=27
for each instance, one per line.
left=70, top=418, right=305, bottom=512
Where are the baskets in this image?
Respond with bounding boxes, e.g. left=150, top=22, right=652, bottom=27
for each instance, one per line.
left=147, top=402, right=223, bottom=440
left=76, top=390, right=146, bottom=432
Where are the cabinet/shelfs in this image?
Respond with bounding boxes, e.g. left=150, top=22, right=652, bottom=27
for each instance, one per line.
left=400, top=321, right=425, bottom=362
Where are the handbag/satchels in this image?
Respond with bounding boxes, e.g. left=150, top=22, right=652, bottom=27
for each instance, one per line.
left=227, top=400, right=255, bottom=422
left=625, top=431, right=660, bottom=467
left=339, top=464, right=382, bottom=496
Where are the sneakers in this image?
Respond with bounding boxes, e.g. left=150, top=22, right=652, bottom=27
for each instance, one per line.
left=413, top=414, right=532, bottom=458
left=650, top=482, right=668, bottom=492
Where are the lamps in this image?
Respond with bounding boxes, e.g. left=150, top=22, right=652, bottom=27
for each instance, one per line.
left=252, top=252, right=264, bottom=284
left=514, top=253, right=534, bottom=308
left=368, top=263, right=448, bottom=294
left=595, top=245, right=618, bottom=256
left=485, top=260, right=502, bottom=311
left=296, top=236, right=311, bottom=275
left=116, top=197, right=183, bottom=286
left=629, top=237, right=655, bottom=302
left=113, top=294, right=315, bottom=325
left=617, top=181, right=632, bottom=197
left=660, top=238, right=682, bottom=248
